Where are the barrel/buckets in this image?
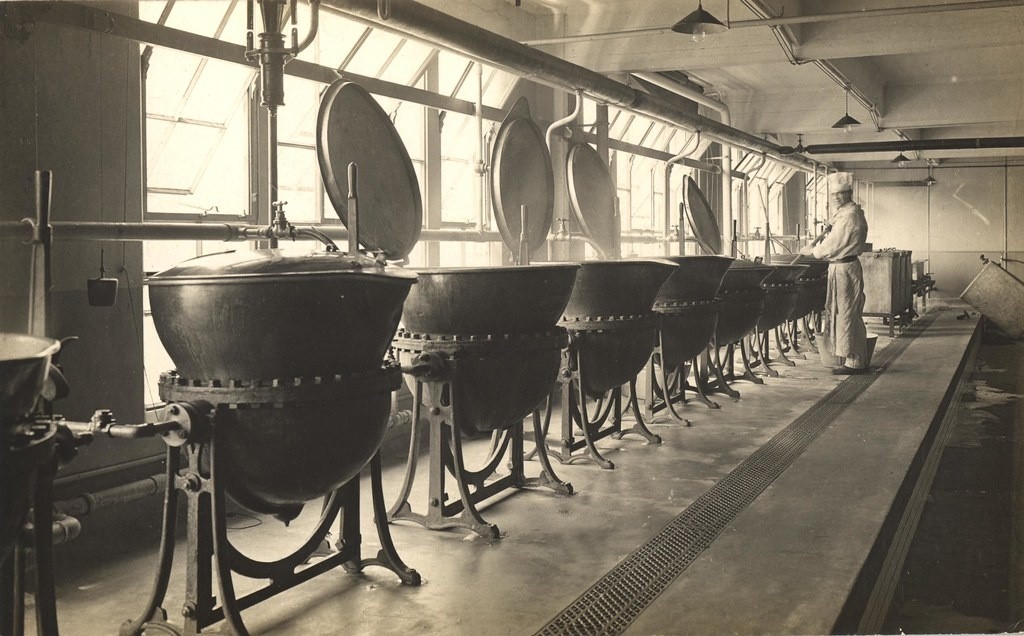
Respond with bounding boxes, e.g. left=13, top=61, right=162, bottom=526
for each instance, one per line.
left=815, top=332, right=878, bottom=367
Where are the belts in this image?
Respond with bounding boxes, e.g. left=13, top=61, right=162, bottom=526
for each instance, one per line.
left=828, top=256, right=853, bottom=263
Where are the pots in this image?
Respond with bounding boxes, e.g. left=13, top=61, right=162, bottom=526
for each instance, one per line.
left=400, top=267, right=580, bottom=339
left=638, top=254, right=736, bottom=301
left=770, top=261, right=828, bottom=283
left=548, top=261, right=680, bottom=319
left=723, top=261, right=775, bottom=300
left=0, top=334, right=59, bottom=433
left=767, top=264, right=808, bottom=282
left=146, top=250, right=417, bottom=379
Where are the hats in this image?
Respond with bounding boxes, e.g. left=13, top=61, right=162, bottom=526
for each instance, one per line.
left=827, top=170, right=857, bottom=193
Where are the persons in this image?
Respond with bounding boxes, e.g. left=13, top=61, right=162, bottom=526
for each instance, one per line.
left=798, top=171, right=870, bottom=375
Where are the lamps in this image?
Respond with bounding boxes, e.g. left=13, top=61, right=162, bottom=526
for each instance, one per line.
left=890, top=137, right=912, bottom=167
left=831, top=85, right=863, bottom=133
left=670, top=0, right=729, bottom=43
left=922, top=166, right=936, bottom=185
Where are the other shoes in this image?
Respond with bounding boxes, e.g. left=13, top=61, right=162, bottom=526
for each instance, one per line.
left=832, top=366, right=870, bottom=377
